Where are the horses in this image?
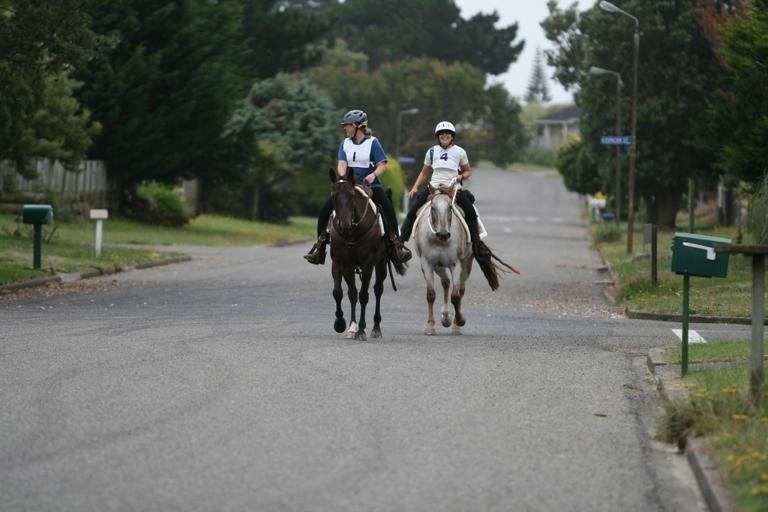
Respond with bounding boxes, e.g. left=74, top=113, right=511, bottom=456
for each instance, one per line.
left=325, top=166, right=411, bottom=342
left=412, top=177, right=522, bottom=336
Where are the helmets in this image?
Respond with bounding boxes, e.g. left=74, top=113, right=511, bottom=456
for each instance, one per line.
left=435, top=121, right=456, bottom=134
left=339, top=110, right=368, bottom=129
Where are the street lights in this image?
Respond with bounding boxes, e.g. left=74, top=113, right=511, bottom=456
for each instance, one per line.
left=600, top=0, right=640, bottom=254
left=589, top=66, right=621, bottom=228
left=395, top=107, right=419, bottom=157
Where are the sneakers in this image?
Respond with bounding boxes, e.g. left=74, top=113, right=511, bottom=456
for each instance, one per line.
left=473, top=242, right=491, bottom=260
left=391, top=236, right=412, bottom=264
left=304, top=248, right=327, bottom=264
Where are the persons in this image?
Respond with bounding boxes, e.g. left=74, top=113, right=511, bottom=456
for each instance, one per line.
left=393, top=120, right=492, bottom=261
left=302, top=109, right=413, bottom=265
